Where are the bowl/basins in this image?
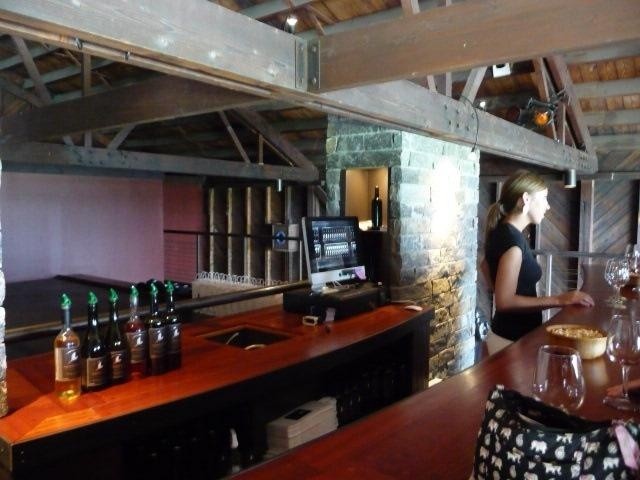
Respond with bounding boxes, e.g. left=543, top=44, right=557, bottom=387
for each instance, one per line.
left=545, top=324, right=608, bottom=360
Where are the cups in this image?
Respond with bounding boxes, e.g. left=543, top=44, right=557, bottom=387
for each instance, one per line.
left=603, top=317, right=640, bottom=412
left=604, top=258, right=629, bottom=304
left=531, top=345, right=586, bottom=416
left=623, top=244, right=640, bottom=291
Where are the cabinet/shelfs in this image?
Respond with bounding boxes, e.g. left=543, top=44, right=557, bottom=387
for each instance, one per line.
left=0, top=299, right=435, bottom=480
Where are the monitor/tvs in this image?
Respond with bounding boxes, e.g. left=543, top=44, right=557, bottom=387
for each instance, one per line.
left=301, top=216, right=366, bottom=284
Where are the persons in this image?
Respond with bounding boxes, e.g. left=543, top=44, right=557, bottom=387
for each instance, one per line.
left=484, top=167, right=595, bottom=357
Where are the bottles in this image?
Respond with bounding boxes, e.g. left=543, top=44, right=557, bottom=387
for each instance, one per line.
left=146, top=279, right=167, bottom=373
left=126, top=283, right=145, bottom=373
left=106, top=287, right=125, bottom=382
left=81, top=291, right=104, bottom=391
left=55, top=294, right=81, bottom=403
left=372, top=184, right=383, bottom=229
left=165, top=281, right=182, bottom=370
